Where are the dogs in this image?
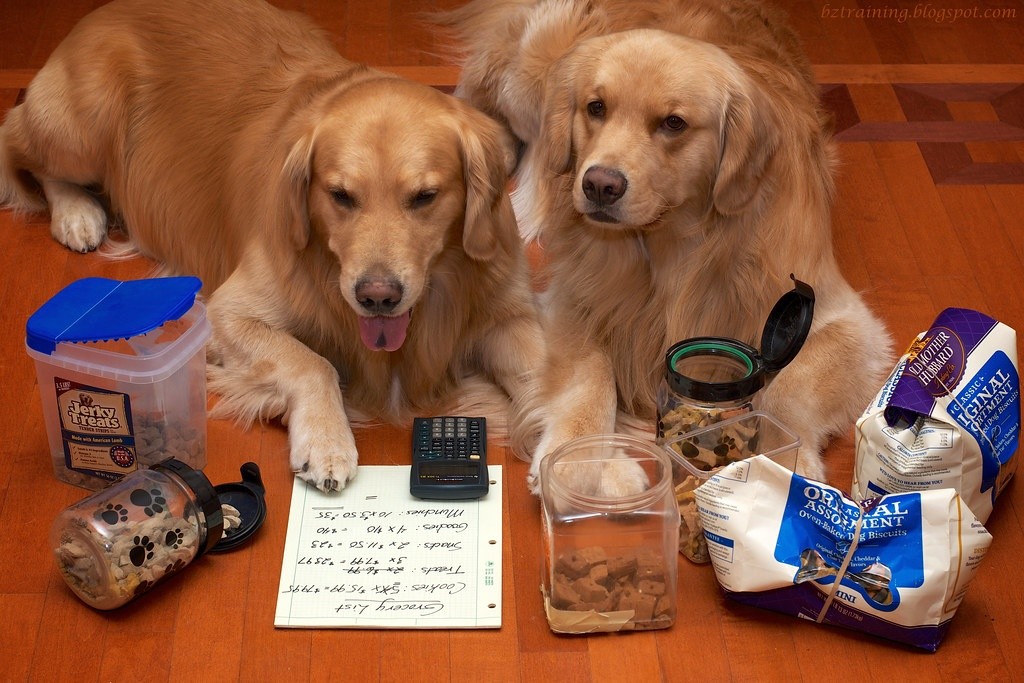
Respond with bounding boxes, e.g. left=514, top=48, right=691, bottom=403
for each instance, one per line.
left=454, top=0, right=898, bottom=531
left=0, top=1, right=650, bottom=526
